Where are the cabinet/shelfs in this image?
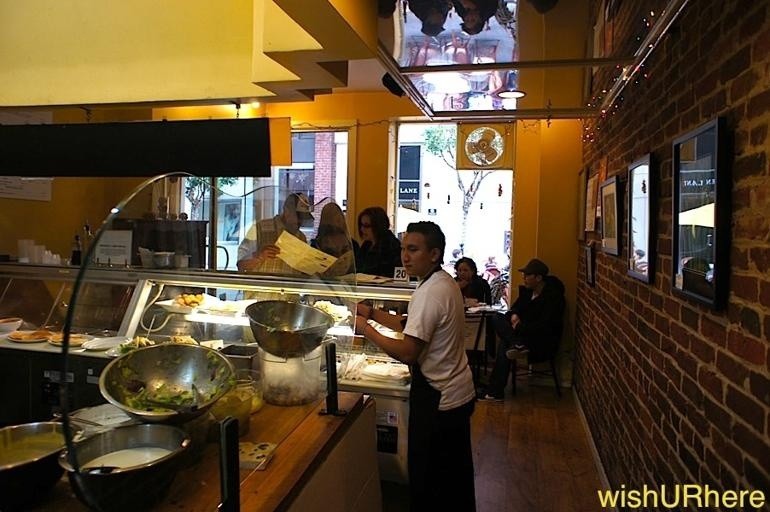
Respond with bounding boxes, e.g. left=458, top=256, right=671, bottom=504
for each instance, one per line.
left=0, top=252, right=419, bottom=477
left=0, top=380, right=383, bottom=512
left=115, top=218, right=209, bottom=271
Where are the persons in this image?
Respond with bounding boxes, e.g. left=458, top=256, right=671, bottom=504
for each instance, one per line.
left=359, top=206, right=402, bottom=276
left=309, top=202, right=359, bottom=279
left=453, top=256, right=492, bottom=304
left=237, top=193, right=314, bottom=276
left=294, top=225, right=356, bottom=317
left=474, top=260, right=565, bottom=402
left=450, top=249, right=462, bottom=264
left=348, top=221, right=478, bottom=511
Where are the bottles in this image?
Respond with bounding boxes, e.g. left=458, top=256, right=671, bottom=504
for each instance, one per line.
left=71, top=234, right=82, bottom=265
left=81, top=219, right=95, bottom=266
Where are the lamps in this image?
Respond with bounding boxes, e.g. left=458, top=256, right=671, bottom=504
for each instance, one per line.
left=408, top=35, right=525, bottom=111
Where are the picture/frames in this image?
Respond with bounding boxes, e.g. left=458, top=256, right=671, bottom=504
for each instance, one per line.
left=600, top=174, right=622, bottom=256
left=575, top=154, right=608, bottom=286
left=628, top=152, right=656, bottom=282
left=672, top=114, right=728, bottom=309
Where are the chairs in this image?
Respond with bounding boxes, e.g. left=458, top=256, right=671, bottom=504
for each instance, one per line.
left=512, top=315, right=567, bottom=401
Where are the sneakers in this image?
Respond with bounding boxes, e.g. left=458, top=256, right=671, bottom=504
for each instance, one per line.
left=476, top=391, right=504, bottom=402
left=505, top=345, right=529, bottom=360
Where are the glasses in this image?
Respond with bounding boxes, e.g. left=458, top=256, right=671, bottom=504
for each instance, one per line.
left=361, top=223, right=371, bottom=228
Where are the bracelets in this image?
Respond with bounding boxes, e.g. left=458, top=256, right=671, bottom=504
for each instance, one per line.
left=368, top=307, right=373, bottom=321
left=358, top=322, right=370, bottom=337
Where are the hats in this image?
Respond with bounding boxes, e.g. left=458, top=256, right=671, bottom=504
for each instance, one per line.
left=285, top=193, right=314, bottom=220
left=517, top=259, right=548, bottom=276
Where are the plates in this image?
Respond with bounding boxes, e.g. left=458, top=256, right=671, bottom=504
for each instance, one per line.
left=5, top=328, right=56, bottom=343
left=48, top=333, right=93, bottom=347
left=104, top=347, right=122, bottom=357
left=153, top=297, right=195, bottom=314
left=81, top=335, right=126, bottom=350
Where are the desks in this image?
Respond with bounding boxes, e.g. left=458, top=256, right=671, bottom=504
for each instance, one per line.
left=460, top=303, right=510, bottom=381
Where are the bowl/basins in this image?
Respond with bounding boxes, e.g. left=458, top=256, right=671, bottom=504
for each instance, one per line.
left=0, top=418, right=86, bottom=496
left=152, top=251, right=175, bottom=267
left=0, top=317, right=25, bottom=333
left=244, top=300, right=334, bottom=359
left=58, top=424, right=190, bottom=505
left=97, top=340, right=235, bottom=419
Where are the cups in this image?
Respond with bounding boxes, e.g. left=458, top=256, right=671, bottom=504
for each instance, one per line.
left=17, top=238, right=61, bottom=264
